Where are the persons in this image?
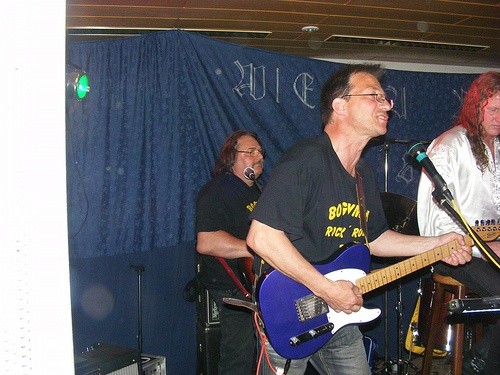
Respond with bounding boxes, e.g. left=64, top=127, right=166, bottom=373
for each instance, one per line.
left=245, top=63, right=472, bottom=375
left=195, top=130, right=265, bottom=375
left=417, top=71, right=500, bottom=375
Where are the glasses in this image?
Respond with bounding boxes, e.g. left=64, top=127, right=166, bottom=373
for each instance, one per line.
left=235, top=146, right=265, bottom=157
left=338, top=93, right=394, bottom=109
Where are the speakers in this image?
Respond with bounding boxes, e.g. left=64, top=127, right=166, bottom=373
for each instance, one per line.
left=195, top=317, right=261, bottom=375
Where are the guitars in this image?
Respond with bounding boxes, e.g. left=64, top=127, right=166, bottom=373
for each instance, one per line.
left=238, top=237, right=259, bottom=294
left=255, top=220, right=500, bottom=359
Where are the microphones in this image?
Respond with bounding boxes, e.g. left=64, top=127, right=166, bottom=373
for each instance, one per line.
left=242, top=167, right=256, bottom=179
left=406, top=139, right=453, bottom=201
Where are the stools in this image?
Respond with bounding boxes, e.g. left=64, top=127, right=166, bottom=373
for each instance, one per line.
left=422, top=273, right=484, bottom=375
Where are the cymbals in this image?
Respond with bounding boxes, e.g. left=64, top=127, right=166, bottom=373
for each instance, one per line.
left=379, top=190, right=430, bottom=231
left=366, top=132, right=432, bottom=149
